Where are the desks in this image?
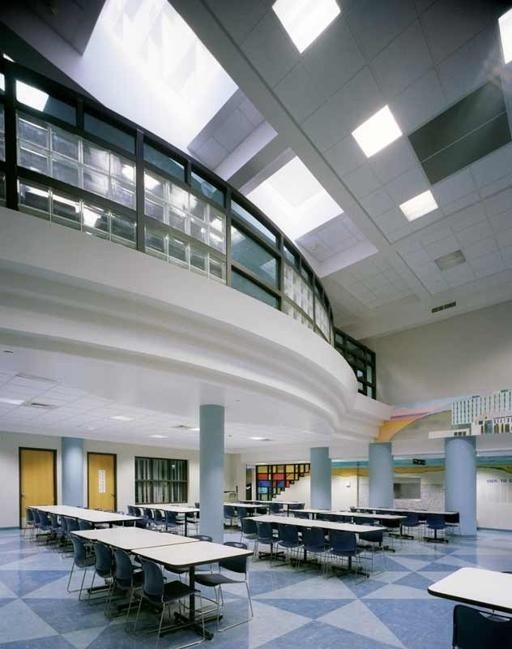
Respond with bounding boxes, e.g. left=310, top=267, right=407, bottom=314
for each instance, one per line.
left=131, top=541, right=254, bottom=641
left=195, top=501, right=267, bottom=528
left=246, top=515, right=386, bottom=578
left=94, top=534, right=199, bottom=612
left=130, top=504, right=199, bottom=537
left=240, top=499, right=305, bottom=517
left=69, top=526, right=160, bottom=593
left=29, top=506, right=143, bottom=547
left=427, top=565, right=512, bottom=620
left=353, top=507, right=459, bottom=543
left=290, top=509, right=408, bottom=523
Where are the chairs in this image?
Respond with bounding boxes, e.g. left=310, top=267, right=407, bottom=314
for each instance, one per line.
left=133, top=554, right=205, bottom=649
left=107, top=548, right=171, bottom=622
left=194, top=541, right=253, bottom=632
left=287, top=504, right=305, bottom=510
left=426, top=514, right=445, bottom=531
left=24, top=507, right=34, bottom=535
left=222, top=504, right=237, bottom=518
left=356, top=523, right=382, bottom=574
left=276, top=522, right=304, bottom=569
left=355, top=517, right=374, bottom=526
left=131, top=506, right=141, bottom=517
left=240, top=518, right=258, bottom=557
left=244, top=501, right=254, bottom=512
left=402, top=514, right=420, bottom=526
left=78, top=520, right=92, bottom=530
left=60, top=517, right=66, bottom=533
left=452, top=604, right=510, bottom=648
left=319, top=514, right=343, bottom=522
left=195, top=502, right=200, bottom=518
left=237, top=507, right=247, bottom=518
left=174, top=510, right=182, bottom=521
left=293, top=510, right=309, bottom=520
left=38, top=510, right=49, bottom=531
left=255, top=502, right=267, bottom=514
left=297, top=526, right=331, bottom=577
left=65, top=518, right=76, bottom=533
left=124, top=514, right=133, bottom=527
left=31, top=509, right=40, bottom=528
left=142, top=507, right=154, bottom=521
left=186, top=507, right=198, bottom=523
left=272, top=503, right=281, bottom=514
left=139, top=531, right=178, bottom=564
left=50, top=513, right=60, bottom=529
left=66, top=533, right=107, bottom=600
left=126, top=505, right=131, bottom=515
left=113, top=511, right=124, bottom=526
left=164, top=509, right=183, bottom=527
left=136, top=516, right=147, bottom=528
left=254, top=520, right=282, bottom=568
left=88, top=543, right=143, bottom=603
left=161, top=534, right=213, bottom=611
left=153, top=507, right=164, bottom=525
left=327, top=528, right=367, bottom=581
